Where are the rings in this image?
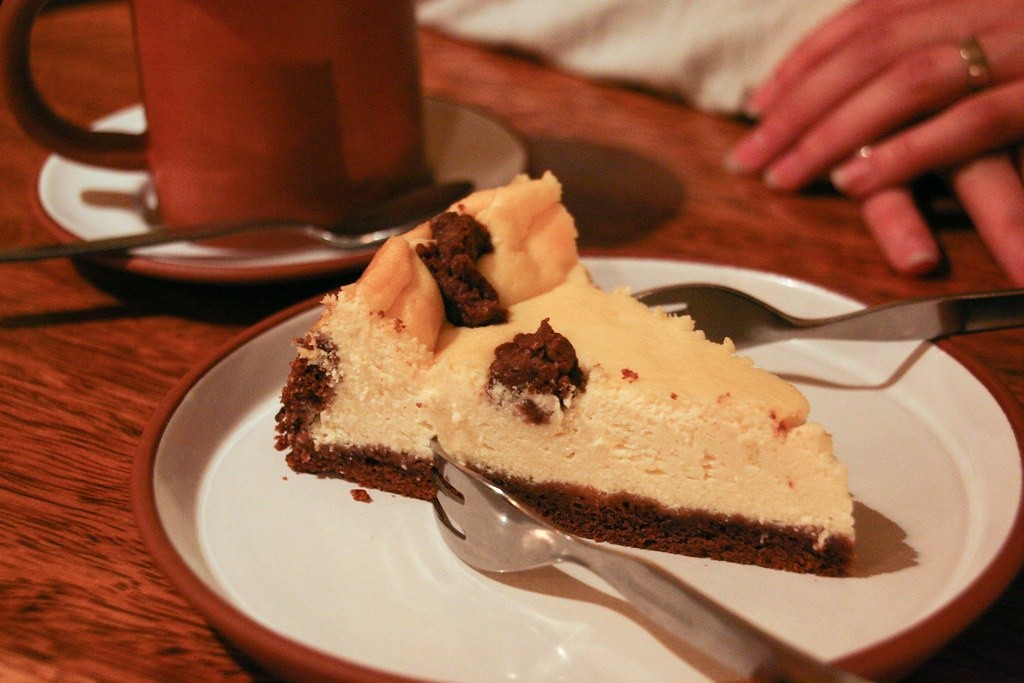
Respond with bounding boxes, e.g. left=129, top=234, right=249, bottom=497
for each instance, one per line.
left=959, top=36, right=992, bottom=93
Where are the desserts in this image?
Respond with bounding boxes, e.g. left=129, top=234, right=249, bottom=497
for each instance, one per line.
left=278, top=172, right=855, bottom=577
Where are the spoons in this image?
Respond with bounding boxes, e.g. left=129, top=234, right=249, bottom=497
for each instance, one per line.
left=0, top=179, right=476, bottom=270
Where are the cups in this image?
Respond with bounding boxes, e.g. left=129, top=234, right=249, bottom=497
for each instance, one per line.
left=0, top=1, right=427, bottom=249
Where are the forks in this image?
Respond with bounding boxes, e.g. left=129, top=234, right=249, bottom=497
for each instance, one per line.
left=629, top=282, right=1023, bottom=350
left=429, top=434, right=871, bottom=683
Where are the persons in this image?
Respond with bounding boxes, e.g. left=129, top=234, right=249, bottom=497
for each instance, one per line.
left=412, top=1, right=1024, bottom=284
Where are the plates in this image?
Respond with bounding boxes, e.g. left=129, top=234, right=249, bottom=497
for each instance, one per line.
left=129, top=248, right=1022, bottom=683
left=30, top=91, right=527, bottom=288
